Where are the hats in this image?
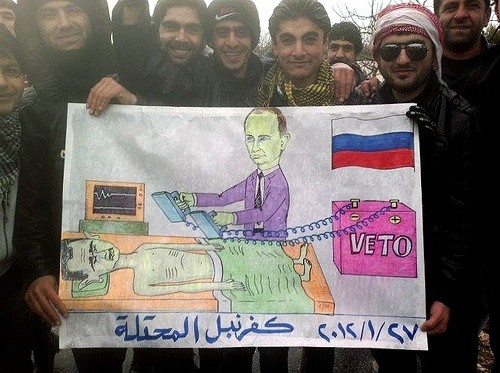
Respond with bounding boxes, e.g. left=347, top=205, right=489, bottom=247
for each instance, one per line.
left=371, top=3, right=449, bottom=86
left=206, top=0, right=261, bottom=50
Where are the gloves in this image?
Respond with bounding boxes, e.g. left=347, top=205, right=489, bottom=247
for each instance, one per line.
left=406, top=106, right=438, bottom=164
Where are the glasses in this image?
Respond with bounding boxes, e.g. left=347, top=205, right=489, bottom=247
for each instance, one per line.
left=379, top=42, right=430, bottom=62
left=0, top=62, right=25, bottom=79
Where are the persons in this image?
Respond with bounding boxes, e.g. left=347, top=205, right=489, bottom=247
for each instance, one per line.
left=358, top=5, right=500, bottom=373
left=1, top=2, right=500, bottom=373
